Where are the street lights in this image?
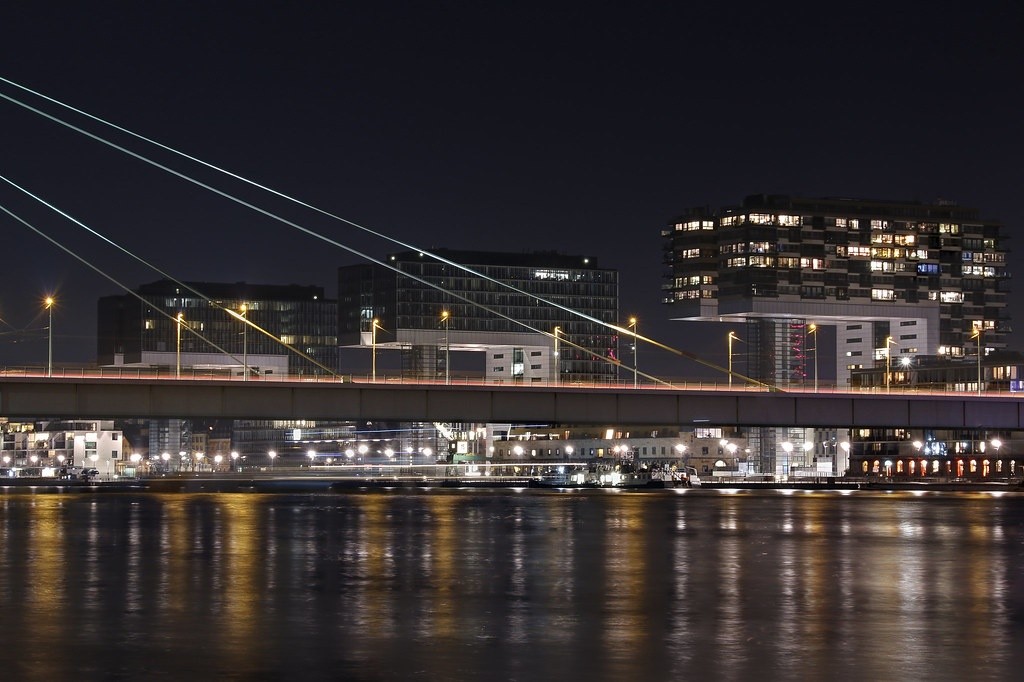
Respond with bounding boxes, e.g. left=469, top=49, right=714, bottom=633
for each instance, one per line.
left=887, top=336, right=893, bottom=394
left=442, top=311, right=450, bottom=386
left=630, top=315, right=638, bottom=390
left=241, top=304, right=249, bottom=380
left=1, top=434, right=1006, bottom=478
left=810, top=323, right=818, bottom=392
left=177, top=312, right=184, bottom=378
left=372, top=318, right=380, bottom=379
left=45, top=297, right=56, bottom=377
left=729, top=331, right=734, bottom=390
left=974, top=330, right=981, bottom=397
left=553, top=325, right=560, bottom=385
